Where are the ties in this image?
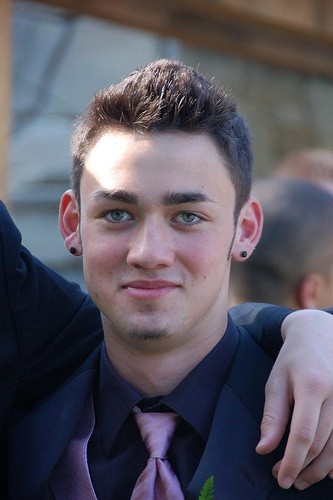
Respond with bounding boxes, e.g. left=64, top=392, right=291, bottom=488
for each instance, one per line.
left=130, top=407, right=185, bottom=500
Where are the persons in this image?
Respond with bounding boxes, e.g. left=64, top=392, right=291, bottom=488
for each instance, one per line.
left=0, top=57, right=333, bottom=500
